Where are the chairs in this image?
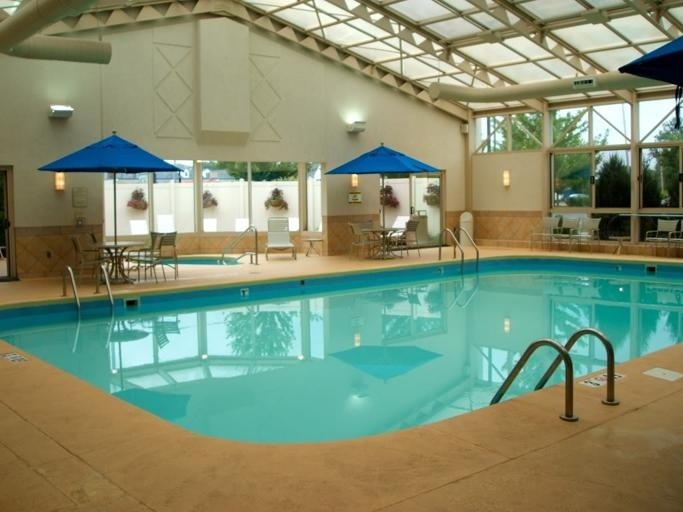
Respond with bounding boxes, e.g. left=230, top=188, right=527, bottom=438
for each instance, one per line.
left=528, top=214, right=601, bottom=256
left=644, top=219, right=682, bottom=257
left=71, top=230, right=179, bottom=288
left=265, top=216, right=297, bottom=262
left=346, top=219, right=420, bottom=259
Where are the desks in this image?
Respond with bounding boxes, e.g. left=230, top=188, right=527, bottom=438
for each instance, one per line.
left=608, top=235, right=631, bottom=256
left=302, top=239, right=323, bottom=257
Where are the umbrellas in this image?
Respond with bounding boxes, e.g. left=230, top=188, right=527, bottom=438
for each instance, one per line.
left=618, top=34, right=682, bottom=88
left=109, top=321, right=191, bottom=422
left=37, top=129, right=186, bottom=278
left=323, top=292, right=443, bottom=383
left=324, top=141, right=444, bottom=254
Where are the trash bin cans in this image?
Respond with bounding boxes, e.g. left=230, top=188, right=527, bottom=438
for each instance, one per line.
left=460, top=212, right=473, bottom=247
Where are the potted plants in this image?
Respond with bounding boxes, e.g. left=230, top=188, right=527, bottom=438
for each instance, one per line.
left=264, top=187, right=289, bottom=210
